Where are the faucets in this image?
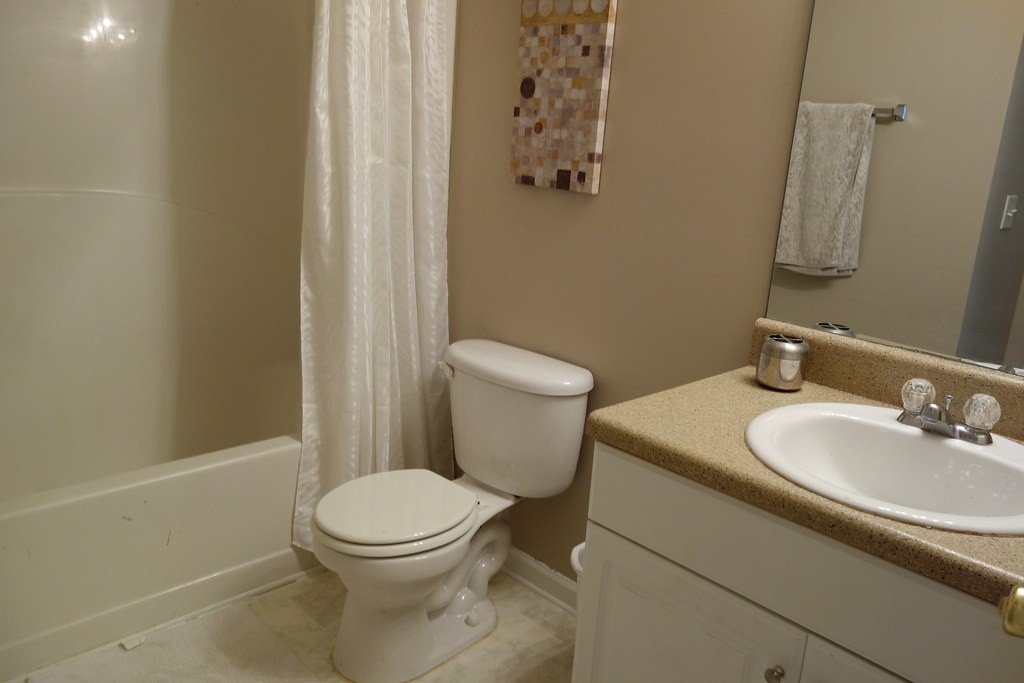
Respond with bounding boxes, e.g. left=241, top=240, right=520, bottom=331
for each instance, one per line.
left=896, top=379, right=1001, bottom=446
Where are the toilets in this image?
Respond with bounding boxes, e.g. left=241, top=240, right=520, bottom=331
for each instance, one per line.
left=311, top=339, right=593, bottom=683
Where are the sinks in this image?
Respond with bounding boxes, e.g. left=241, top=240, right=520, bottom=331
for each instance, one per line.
left=744, top=402, right=1024, bottom=537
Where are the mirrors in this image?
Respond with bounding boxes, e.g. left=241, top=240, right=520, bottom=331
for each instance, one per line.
left=764, top=0, right=1024, bottom=379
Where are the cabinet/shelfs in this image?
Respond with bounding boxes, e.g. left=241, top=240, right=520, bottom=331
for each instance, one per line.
left=571, top=441, right=1024, bottom=683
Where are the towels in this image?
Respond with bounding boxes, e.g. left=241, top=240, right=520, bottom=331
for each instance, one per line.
left=773, top=100, right=878, bottom=278
